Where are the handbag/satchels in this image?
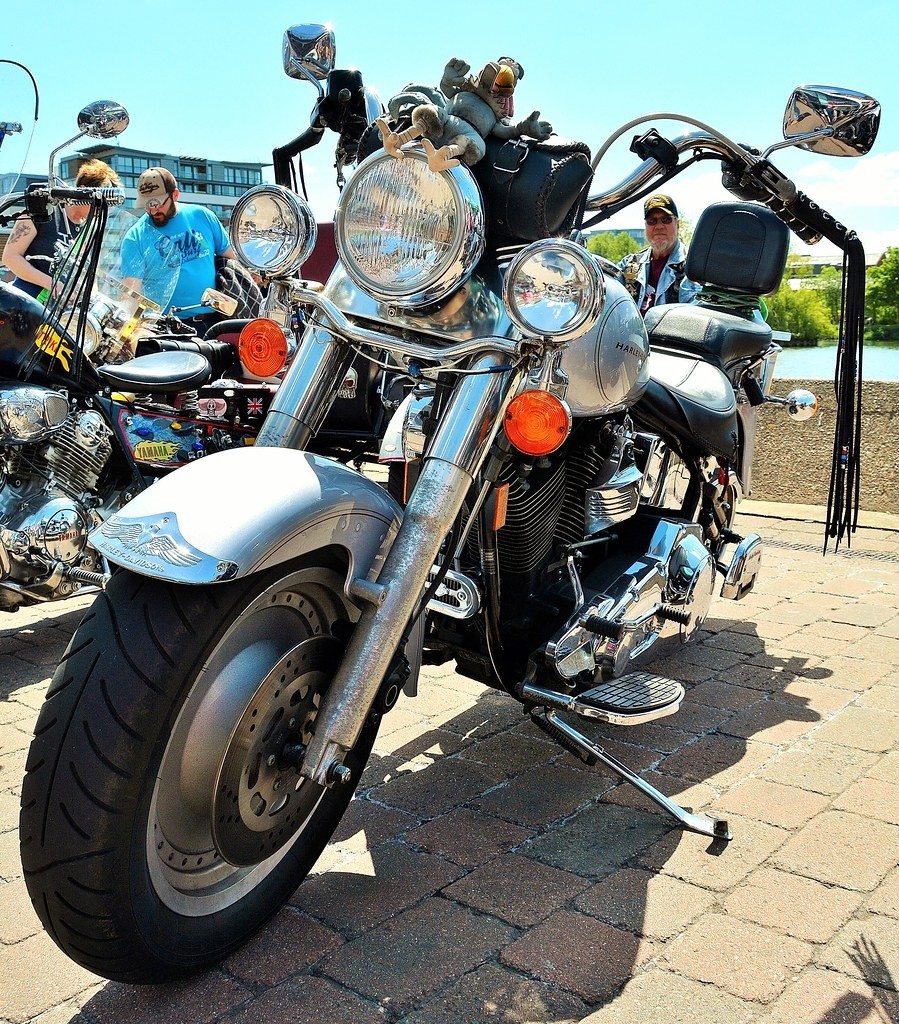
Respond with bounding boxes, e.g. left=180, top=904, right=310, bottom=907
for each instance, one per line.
left=355, top=108, right=594, bottom=240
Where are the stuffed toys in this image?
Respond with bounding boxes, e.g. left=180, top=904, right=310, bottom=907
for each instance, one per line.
left=375, top=55, right=551, bottom=172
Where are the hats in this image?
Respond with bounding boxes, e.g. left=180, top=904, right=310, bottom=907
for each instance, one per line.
left=135, top=167, right=179, bottom=208
left=644, top=194, right=678, bottom=220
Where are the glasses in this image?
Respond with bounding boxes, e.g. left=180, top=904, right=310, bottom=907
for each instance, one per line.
left=647, top=215, right=673, bottom=224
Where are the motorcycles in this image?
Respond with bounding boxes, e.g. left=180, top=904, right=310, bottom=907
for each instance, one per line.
left=16, top=24, right=881, bottom=992
left=0, top=58, right=395, bottom=615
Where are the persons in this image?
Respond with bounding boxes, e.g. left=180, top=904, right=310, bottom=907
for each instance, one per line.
left=616, top=194, right=702, bottom=319
left=3, top=159, right=124, bottom=309
left=119, top=167, right=238, bottom=339
left=822, top=100, right=880, bottom=153
left=787, top=94, right=797, bottom=125
